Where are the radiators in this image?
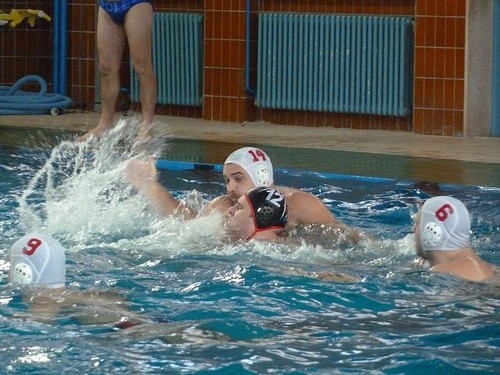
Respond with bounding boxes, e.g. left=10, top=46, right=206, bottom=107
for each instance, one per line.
left=129, top=12, right=204, bottom=107
left=256, top=12, right=415, bottom=117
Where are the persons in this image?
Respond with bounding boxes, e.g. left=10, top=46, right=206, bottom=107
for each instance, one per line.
left=124, top=146, right=370, bottom=249
left=4, top=233, right=235, bottom=348
left=413, top=196, right=500, bottom=284
left=74, top=0, right=158, bottom=146
